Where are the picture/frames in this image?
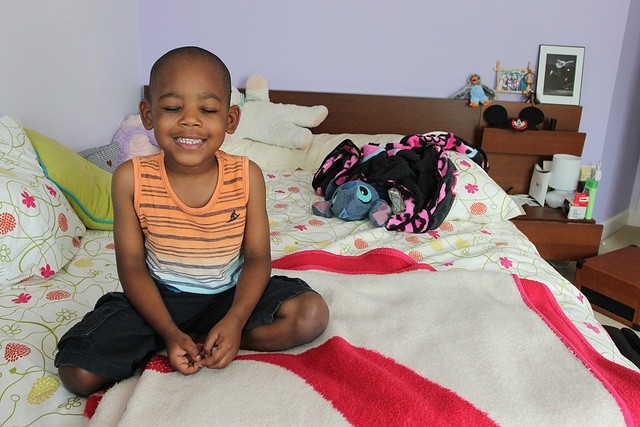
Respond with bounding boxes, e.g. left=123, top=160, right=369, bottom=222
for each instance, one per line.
left=494, top=60, right=531, bottom=102
left=536, top=44, right=585, bottom=106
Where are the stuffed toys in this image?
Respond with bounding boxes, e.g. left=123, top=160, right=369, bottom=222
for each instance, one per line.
left=454, top=74, right=495, bottom=108
left=230, top=73, right=329, bottom=149
left=113, top=113, right=161, bottom=163
left=312, top=179, right=393, bottom=228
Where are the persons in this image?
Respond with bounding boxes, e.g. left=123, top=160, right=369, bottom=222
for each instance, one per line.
left=54, top=45, right=331, bottom=398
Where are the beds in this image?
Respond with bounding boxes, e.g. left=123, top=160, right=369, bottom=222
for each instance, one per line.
left=0, top=86, right=640, bottom=427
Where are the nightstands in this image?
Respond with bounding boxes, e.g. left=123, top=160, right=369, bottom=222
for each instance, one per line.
left=508, top=195, right=604, bottom=261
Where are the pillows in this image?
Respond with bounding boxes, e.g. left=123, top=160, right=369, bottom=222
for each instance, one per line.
left=0, top=115, right=86, bottom=280
left=299, top=132, right=404, bottom=172
left=219, top=138, right=308, bottom=172
left=25, top=127, right=114, bottom=231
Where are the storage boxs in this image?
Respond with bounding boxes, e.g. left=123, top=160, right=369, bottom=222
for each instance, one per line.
left=575, top=244, right=640, bottom=331
